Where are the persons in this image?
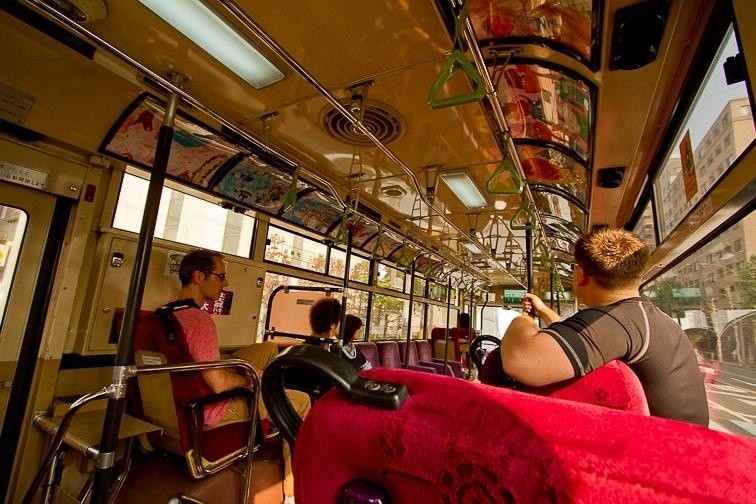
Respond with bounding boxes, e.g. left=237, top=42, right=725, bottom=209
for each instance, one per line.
left=332, top=315, right=373, bottom=373
left=457, top=313, right=477, bottom=339
left=285, top=299, right=342, bottom=397
left=166, top=249, right=312, bottom=504
left=500, top=225, right=709, bottom=428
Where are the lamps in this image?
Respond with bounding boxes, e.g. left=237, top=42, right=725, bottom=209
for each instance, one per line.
left=139, top=0, right=291, bottom=90
left=438, top=168, right=492, bottom=210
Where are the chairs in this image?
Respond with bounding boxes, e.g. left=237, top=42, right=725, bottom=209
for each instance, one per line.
left=114, top=307, right=279, bottom=504
left=257, top=335, right=755, bottom=502
left=429, top=323, right=484, bottom=366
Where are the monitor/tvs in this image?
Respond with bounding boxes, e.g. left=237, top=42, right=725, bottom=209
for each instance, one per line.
left=504, top=289, right=526, bottom=304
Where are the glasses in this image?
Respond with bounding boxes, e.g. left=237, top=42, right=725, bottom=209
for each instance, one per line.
left=571, top=261, right=592, bottom=276
left=205, top=271, right=225, bottom=281
